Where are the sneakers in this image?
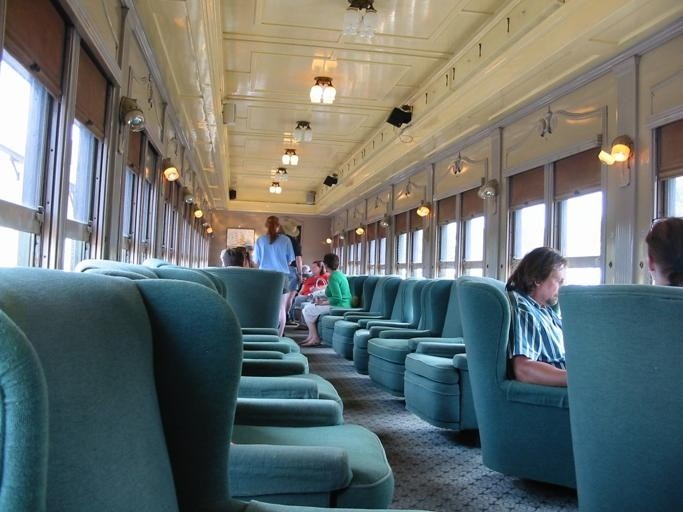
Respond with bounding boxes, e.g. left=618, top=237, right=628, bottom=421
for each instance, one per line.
left=298, top=338, right=320, bottom=346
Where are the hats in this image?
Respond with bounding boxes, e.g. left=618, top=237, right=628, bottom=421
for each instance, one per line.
left=281, top=218, right=298, bottom=237
left=223, top=248, right=242, bottom=266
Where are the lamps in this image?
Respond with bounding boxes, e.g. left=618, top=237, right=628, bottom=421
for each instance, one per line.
left=476, top=180, right=498, bottom=200
left=380, top=213, right=394, bottom=228
left=339, top=0, right=384, bottom=41
left=184, top=189, right=213, bottom=234
left=598, top=134, right=634, bottom=167
left=308, top=77, right=337, bottom=104
left=415, top=199, right=431, bottom=217
left=275, top=121, right=313, bottom=182
left=164, top=157, right=180, bottom=182
left=118, top=95, right=146, bottom=133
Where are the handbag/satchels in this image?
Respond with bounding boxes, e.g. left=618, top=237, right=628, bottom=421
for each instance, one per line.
left=309, top=278, right=327, bottom=293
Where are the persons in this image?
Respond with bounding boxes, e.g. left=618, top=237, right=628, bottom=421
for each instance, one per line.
left=503, top=245, right=570, bottom=388
left=219, top=243, right=255, bottom=268
left=278, top=219, right=303, bottom=324
left=252, top=216, right=296, bottom=340
left=294, top=260, right=330, bottom=329
left=299, top=253, right=351, bottom=347
left=646, top=215, right=682, bottom=286
left=299, top=265, right=311, bottom=287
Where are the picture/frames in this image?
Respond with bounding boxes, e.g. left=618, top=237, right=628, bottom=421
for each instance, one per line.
left=226, top=227, right=256, bottom=253
